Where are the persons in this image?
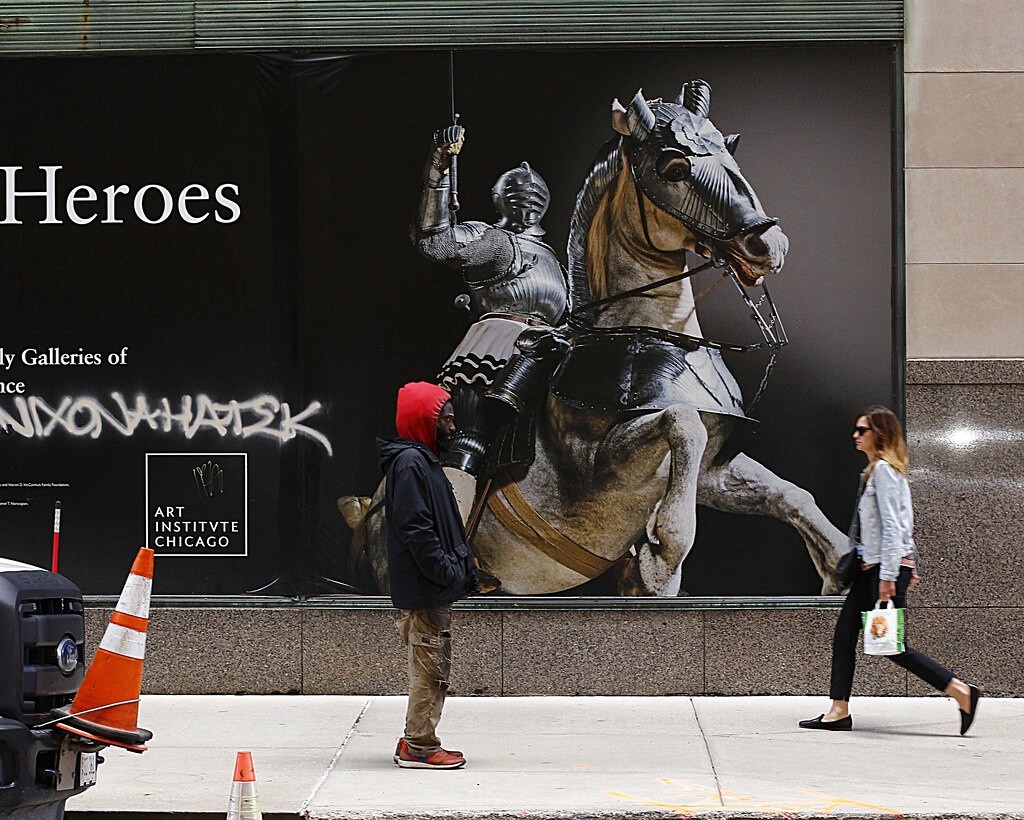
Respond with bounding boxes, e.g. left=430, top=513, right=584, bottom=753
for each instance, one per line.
left=391, top=118, right=571, bottom=475
left=799, top=405, right=980, bottom=734
left=375, top=382, right=483, bottom=767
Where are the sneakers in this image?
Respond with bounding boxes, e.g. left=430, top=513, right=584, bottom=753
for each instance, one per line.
left=397, top=737, right=466, bottom=770
left=390, top=736, right=464, bottom=764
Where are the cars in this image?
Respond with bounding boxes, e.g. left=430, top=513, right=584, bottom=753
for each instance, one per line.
left=2, top=547, right=106, bottom=820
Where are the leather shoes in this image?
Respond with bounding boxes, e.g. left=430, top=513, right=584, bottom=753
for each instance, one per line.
left=958, top=681, right=981, bottom=737
left=798, top=713, right=853, bottom=732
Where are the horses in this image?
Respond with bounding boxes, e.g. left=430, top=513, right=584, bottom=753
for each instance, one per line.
left=338, top=83, right=852, bottom=596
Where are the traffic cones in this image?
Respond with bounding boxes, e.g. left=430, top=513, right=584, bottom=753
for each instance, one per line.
left=223, top=749, right=261, bottom=818
left=58, top=541, right=157, bottom=757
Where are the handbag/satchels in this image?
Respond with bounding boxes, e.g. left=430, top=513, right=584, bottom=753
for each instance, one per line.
left=860, top=597, right=907, bottom=657
left=834, top=548, right=861, bottom=592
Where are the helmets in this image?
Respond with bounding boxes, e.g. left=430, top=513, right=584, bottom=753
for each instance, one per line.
left=491, top=160, right=551, bottom=229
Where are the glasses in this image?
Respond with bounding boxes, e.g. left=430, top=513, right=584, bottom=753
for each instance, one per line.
left=851, top=425, right=872, bottom=437
